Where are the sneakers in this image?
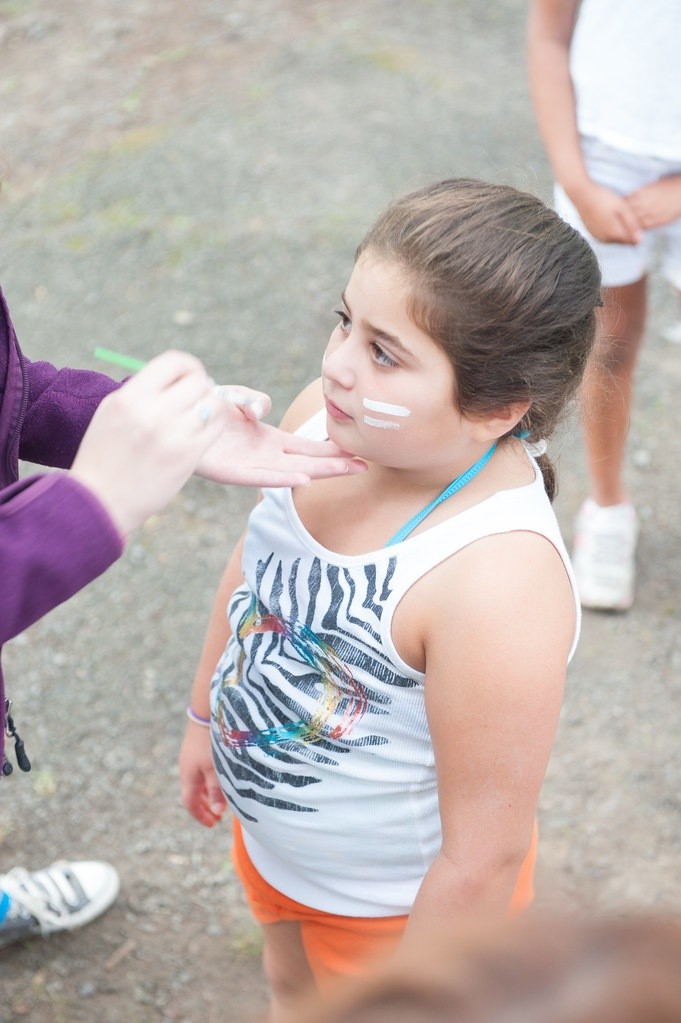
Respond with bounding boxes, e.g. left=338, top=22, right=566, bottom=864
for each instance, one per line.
left=0, top=859, right=120, bottom=951
left=573, top=499, right=638, bottom=611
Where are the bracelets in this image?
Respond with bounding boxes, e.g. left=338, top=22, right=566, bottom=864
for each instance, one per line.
left=186, top=706, right=213, bottom=728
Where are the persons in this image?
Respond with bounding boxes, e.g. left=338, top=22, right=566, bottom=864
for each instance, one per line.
left=525, top=0, right=681, bottom=614
left=1, top=284, right=367, bottom=940
left=287, top=906, right=681, bottom=1023
left=179, top=174, right=606, bottom=1022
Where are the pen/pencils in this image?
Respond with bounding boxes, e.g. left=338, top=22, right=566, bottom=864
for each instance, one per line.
left=93, top=345, right=146, bottom=372
